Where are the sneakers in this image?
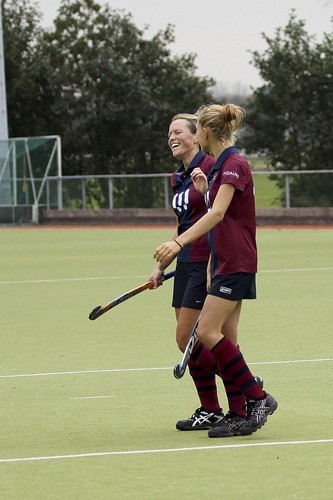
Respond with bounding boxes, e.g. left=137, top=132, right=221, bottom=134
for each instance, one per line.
left=238, top=392, right=278, bottom=435
left=176, top=406, right=225, bottom=430
left=254, top=376, right=264, bottom=389
left=208, top=410, right=247, bottom=437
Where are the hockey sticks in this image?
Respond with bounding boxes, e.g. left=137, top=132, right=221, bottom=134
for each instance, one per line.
left=173, top=314, right=200, bottom=379
left=89, top=270, right=176, bottom=320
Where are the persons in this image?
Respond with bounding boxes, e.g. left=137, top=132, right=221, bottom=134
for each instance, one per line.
left=149, top=113, right=263, bottom=430
left=153, top=104, right=277, bottom=437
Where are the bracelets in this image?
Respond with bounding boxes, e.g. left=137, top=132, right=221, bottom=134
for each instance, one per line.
left=174, top=240, right=183, bottom=250
